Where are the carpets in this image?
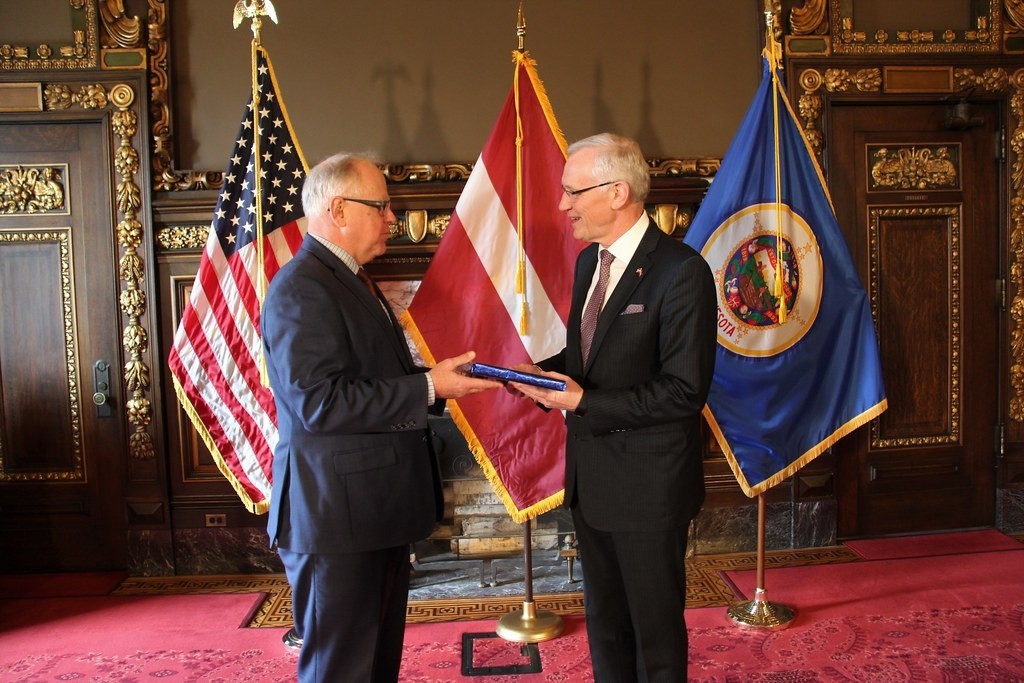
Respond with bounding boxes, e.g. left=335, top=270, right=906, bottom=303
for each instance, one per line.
left=842, top=529, right=1024, bottom=560
left=0, top=570, right=129, bottom=599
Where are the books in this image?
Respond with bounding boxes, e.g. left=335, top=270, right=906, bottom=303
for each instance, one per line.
left=458, top=359, right=568, bottom=395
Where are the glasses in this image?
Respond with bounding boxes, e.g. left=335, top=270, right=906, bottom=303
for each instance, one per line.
left=562, top=181, right=615, bottom=202
left=328, top=196, right=389, bottom=215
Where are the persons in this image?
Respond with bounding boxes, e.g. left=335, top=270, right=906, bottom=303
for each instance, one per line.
left=509, top=133, right=718, bottom=683
left=260, top=152, right=500, bottom=681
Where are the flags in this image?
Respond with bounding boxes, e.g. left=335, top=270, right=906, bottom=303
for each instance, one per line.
left=398, top=50, right=590, bottom=523
left=682, top=25, right=869, bottom=498
left=168, top=45, right=311, bottom=516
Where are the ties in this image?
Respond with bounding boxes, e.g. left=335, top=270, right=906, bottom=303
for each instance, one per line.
left=356, top=266, right=378, bottom=301
left=580, top=250, right=616, bottom=374
